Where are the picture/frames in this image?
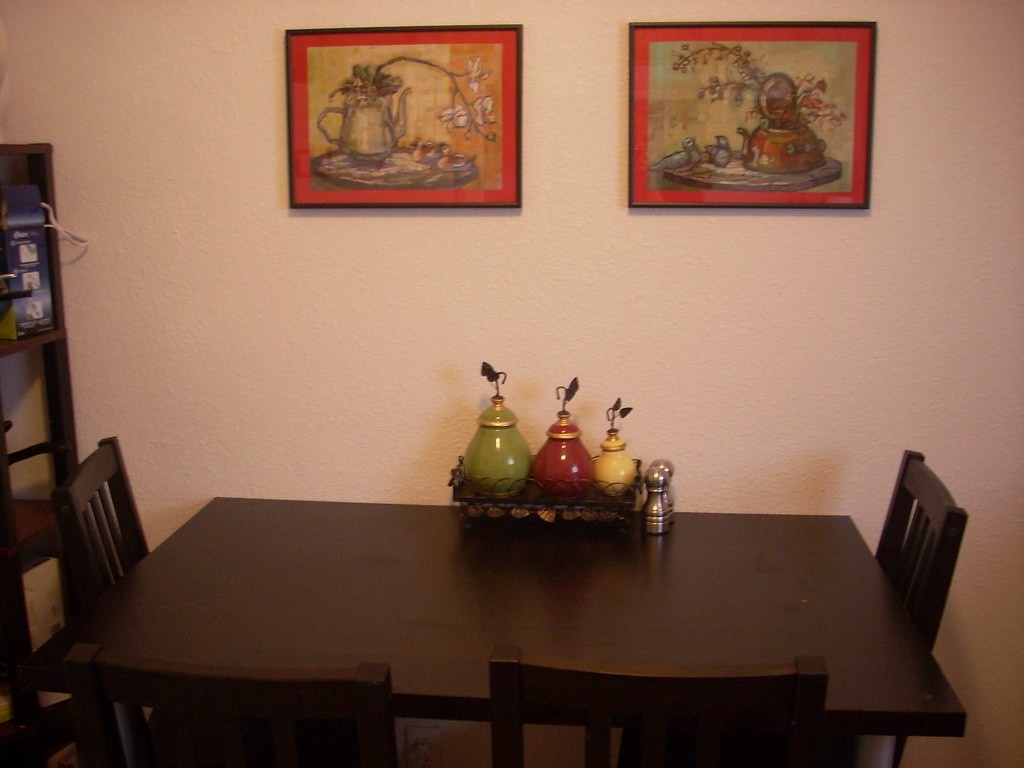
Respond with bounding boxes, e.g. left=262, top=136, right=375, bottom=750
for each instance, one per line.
left=626, top=21, right=876, bottom=211
left=284, top=25, right=525, bottom=208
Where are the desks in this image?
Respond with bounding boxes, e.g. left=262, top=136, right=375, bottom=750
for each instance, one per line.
left=17, top=497, right=970, bottom=768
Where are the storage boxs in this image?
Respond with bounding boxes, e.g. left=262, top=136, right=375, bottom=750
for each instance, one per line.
left=1, top=183, right=53, bottom=339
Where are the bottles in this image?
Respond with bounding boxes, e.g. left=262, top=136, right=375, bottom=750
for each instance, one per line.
left=644, top=468, right=669, bottom=534
left=463, top=361, right=531, bottom=497
left=594, top=398, right=638, bottom=497
left=649, top=460, right=674, bottom=526
left=535, top=377, right=593, bottom=498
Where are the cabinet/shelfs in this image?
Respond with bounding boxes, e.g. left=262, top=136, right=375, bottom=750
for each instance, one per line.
left=0, top=142, right=85, bottom=768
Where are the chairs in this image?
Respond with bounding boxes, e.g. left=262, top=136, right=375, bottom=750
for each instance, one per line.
left=487, top=659, right=830, bottom=768
left=60, top=642, right=400, bottom=768
left=879, top=447, right=968, bottom=657
left=51, top=436, right=149, bottom=603
